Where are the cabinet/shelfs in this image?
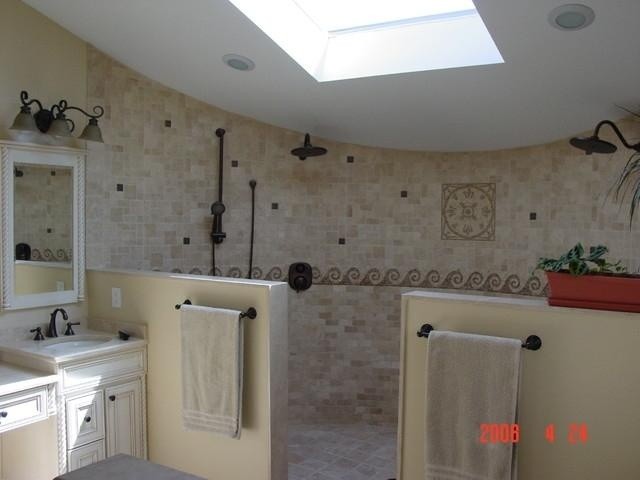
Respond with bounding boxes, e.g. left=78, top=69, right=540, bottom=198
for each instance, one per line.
left=0, top=346, right=149, bottom=480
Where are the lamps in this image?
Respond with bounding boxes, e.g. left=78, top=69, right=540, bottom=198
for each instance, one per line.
left=8, top=90, right=105, bottom=144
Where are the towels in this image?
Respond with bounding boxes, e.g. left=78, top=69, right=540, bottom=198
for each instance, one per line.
left=179, top=305, right=245, bottom=440
left=422, top=329, right=523, bottom=480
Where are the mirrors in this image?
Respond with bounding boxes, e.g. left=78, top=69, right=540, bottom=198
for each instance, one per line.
left=0, top=138, right=86, bottom=311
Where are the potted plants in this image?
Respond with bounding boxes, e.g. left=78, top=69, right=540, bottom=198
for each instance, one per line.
left=528, top=242, right=640, bottom=313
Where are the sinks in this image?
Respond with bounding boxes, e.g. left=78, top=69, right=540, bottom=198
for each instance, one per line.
left=41, top=334, right=112, bottom=352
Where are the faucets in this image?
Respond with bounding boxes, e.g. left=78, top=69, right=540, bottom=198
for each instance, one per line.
left=47, top=308, right=68, bottom=337
